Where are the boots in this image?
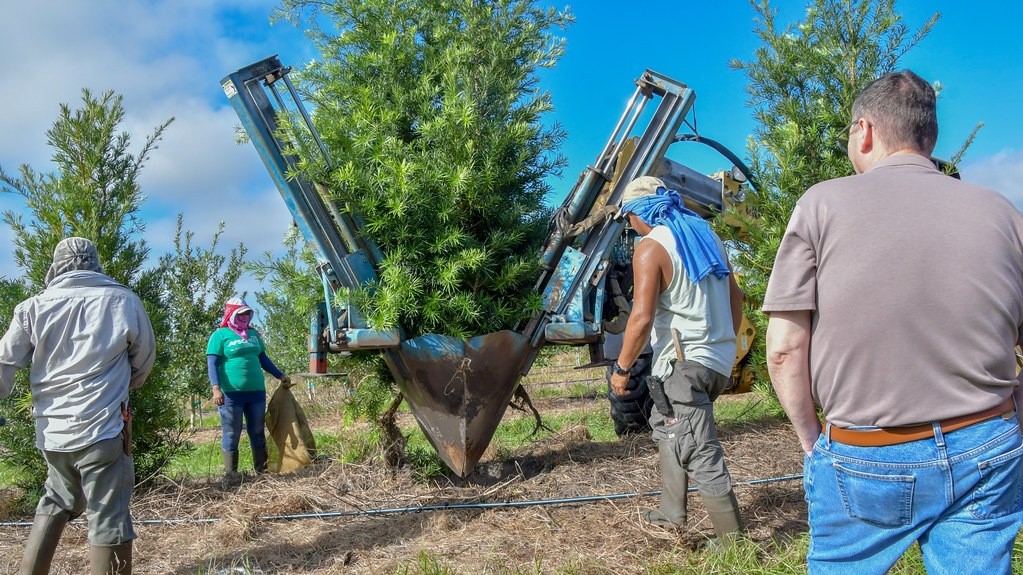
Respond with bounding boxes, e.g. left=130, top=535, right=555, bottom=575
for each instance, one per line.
left=702, top=488, right=745, bottom=553
left=222, top=449, right=238, bottom=475
left=252, top=446, right=268, bottom=476
left=89, top=539, right=132, bottom=575
left=18, top=514, right=67, bottom=575
left=642, top=438, right=688, bottom=530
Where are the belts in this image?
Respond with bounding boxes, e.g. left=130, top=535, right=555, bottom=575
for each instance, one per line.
left=821, top=393, right=1016, bottom=447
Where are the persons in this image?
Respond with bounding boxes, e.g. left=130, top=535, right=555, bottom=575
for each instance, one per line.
left=762, top=69, right=1023, bottom=575
left=0, top=237, right=156, bottom=575
left=207, top=297, right=291, bottom=477
left=610, top=176, right=747, bottom=555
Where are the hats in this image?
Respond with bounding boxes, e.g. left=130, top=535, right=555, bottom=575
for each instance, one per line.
left=227, top=298, right=253, bottom=314
left=613, top=176, right=669, bottom=220
left=45, top=237, right=103, bottom=289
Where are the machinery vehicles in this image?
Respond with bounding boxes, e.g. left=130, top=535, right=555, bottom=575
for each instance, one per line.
left=220, top=51, right=962, bottom=477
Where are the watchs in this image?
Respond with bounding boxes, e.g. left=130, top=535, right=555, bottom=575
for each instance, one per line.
left=614, top=361, right=631, bottom=375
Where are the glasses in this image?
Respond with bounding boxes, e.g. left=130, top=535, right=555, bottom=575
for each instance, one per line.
left=623, top=210, right=631, bottom=225
left=835, top=120, right=874, bottom=158
